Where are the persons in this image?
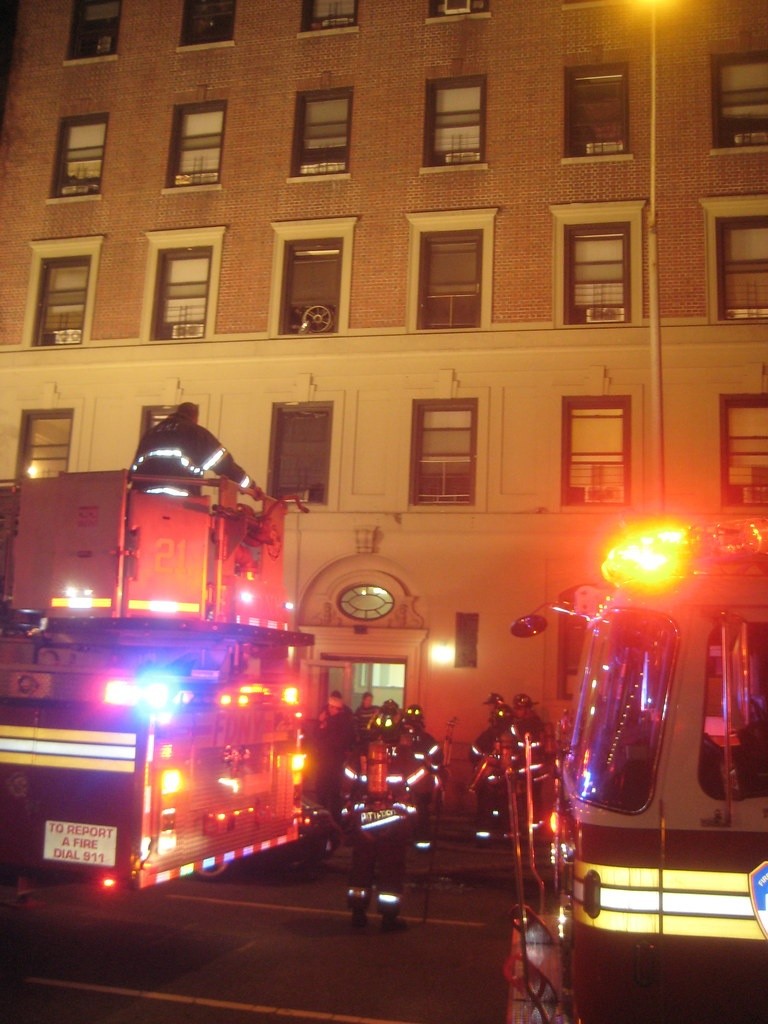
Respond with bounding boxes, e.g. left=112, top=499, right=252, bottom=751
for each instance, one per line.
left=313, top=690, right=550, bottom=931
left=129, top=401, right=264, bottom=503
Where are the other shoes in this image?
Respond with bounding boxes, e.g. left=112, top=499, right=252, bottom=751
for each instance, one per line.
left=470, top=839, right=489, bottom=848
left=418, top=844, right=430, bottom=853
left=380, top=917, right=407, bottom=933
left=351, top=911, right=368, bottom=927
left=494, top=838, right=523, bottom=851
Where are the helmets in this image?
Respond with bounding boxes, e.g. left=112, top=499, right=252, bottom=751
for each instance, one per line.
left=329, top=690, right=345, bottom=708
left=405, top=705, right=423, bottom=718
left=372, top=713, right=394, bottom=730
left=512, top=694, right=538, bottom=708
left=381, top=701, right=398, bottom=714
left=483, top=694, right=504, bottom=706
left=491, top=704, right=513, bottom=720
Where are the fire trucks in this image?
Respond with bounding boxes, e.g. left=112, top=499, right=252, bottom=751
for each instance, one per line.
left=0, top=462, right=320, bottom=918
left=508, top=508, right=768, bottom=1024
